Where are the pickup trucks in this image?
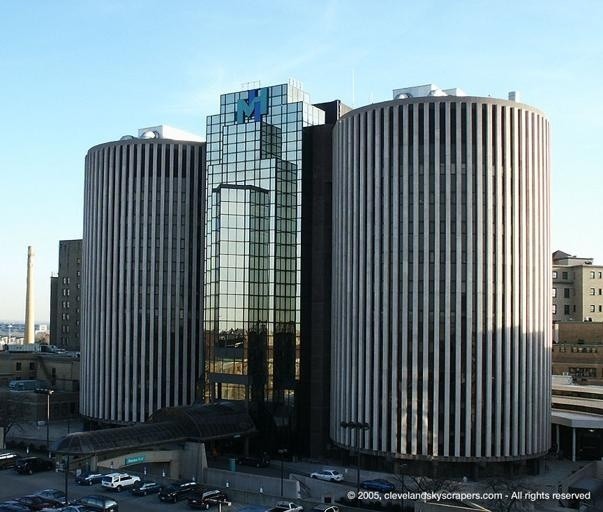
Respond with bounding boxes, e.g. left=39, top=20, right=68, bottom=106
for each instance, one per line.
left=265, top=500, right=303, bottom=511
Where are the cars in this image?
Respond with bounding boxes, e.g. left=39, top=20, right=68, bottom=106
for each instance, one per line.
left=0, top=453, right=17, bottom=469
left=75, top=472, right=103, bottom=486
left=311, top=504, right=339, bottom=512
left=310, top=469, right=343, bottom=483
left=360, top=478, right=394, bottom=492
left=131, top=481, right=161, bottom=497
left=0, top=488, right=119, bottom=511
left=238, top=453, right=271, bottom=468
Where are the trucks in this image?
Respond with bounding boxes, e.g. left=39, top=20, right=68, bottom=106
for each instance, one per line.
left=8, top=380, right=39, bottom=391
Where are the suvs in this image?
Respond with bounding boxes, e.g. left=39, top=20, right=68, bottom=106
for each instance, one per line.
left=188, top=488, right=227, bottom=509
left=157, top=480, right=202, bottom=504
left=15, top=457, right=52, bottom=476
left=102, top=472, right=140, bottom=493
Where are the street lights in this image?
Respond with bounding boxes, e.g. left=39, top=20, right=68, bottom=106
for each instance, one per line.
left=6, top=324, right=12, bottom=342
left=398, top=464, right=407, bottom=510
left=277, top=448, right=289, bottom=498
left=34, top=386, right=55, bottom=450
left=339, top=420, right=370, bottom=494
